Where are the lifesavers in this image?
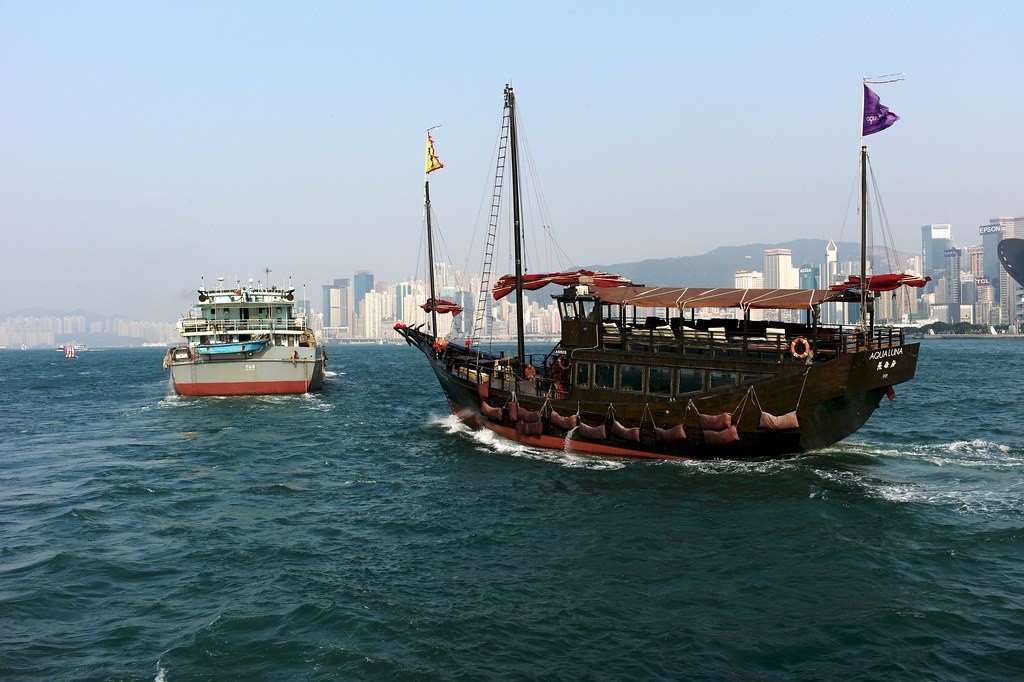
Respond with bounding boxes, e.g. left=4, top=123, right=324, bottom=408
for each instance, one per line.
left=557, top=354, right=573, bottom=370
left=790, top=338, right=811, bottom=359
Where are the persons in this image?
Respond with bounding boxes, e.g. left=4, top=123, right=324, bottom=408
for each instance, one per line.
left=433, top=337, right=449, bottom=360
left=465, top=337, right=471, bottom=356
left=525, top=363, right=536, bottom=380
left=548, top=355, right=570, bottom=379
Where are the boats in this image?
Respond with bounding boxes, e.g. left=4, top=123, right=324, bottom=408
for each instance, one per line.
left=193, top=337, right=271, bottom=355
left=392, top=77, right=932, bottom=461
left=56, top=344, right=86, bottom=358
left=162, top=267, right=328, bottom=397
